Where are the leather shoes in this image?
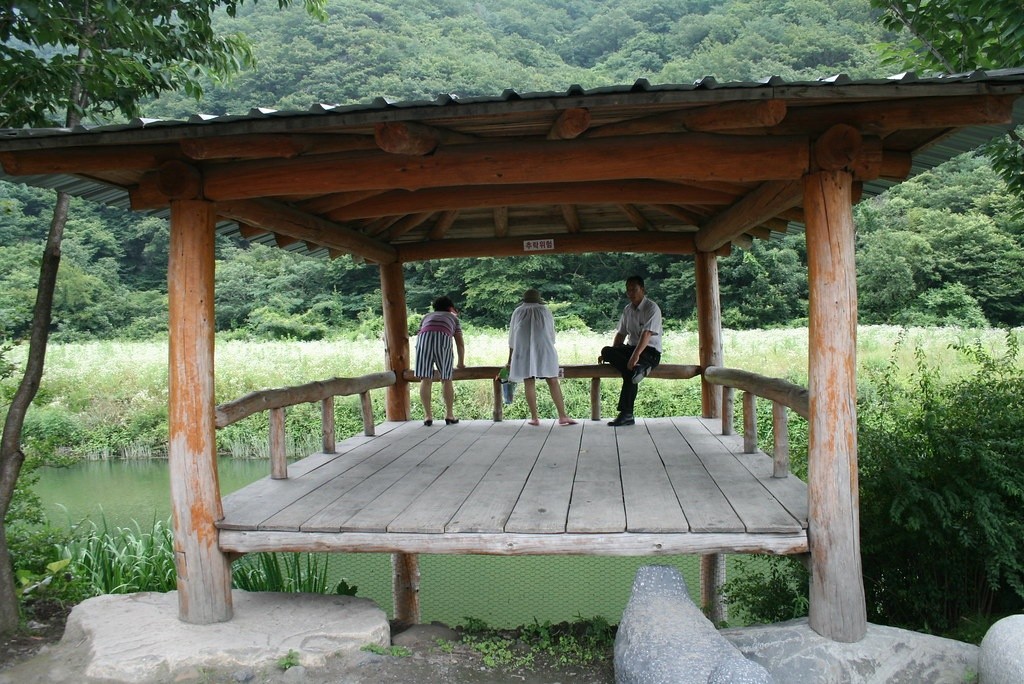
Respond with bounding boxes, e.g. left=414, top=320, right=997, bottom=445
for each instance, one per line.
left=631, top=362, right=653, bottom=384
left=607, top=412, right=635, bottom=426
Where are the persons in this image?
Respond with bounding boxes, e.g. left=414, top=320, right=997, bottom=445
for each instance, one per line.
left=414, top=296, right=465, bottom=426
left=494, top=289, right=578, bottom=425
left=596, top=274, right=661, bottom=426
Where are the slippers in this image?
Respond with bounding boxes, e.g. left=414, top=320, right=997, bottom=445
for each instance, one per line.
left=527, top=419, right=540, bottom=425
left=558, top=417, right=578, bottom=426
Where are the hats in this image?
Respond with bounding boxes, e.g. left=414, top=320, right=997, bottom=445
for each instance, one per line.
left=524, top=290, right=541, bottom=303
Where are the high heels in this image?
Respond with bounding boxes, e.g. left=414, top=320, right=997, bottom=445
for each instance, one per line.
left=445, top=414, right=459, bottom=425
left=423, top=420, right=433, bottom=426
